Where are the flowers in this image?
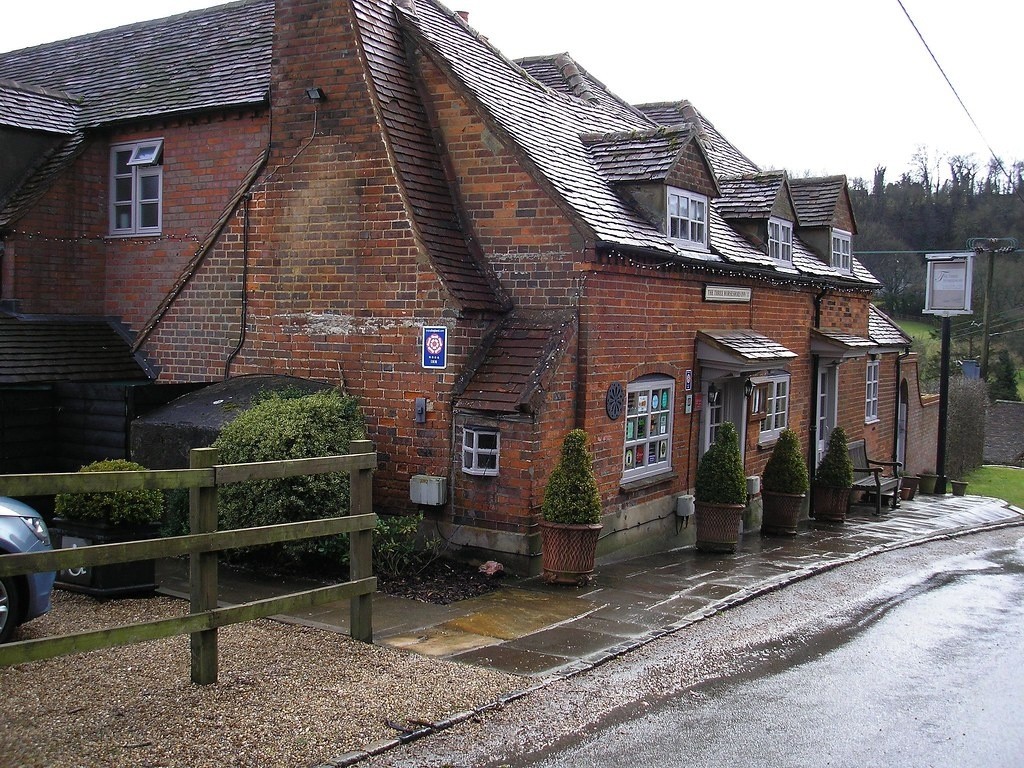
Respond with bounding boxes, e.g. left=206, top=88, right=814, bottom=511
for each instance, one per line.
left=922, top=468, right=935, bottom=475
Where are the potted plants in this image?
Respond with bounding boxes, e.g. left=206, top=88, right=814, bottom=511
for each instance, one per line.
left=813, top=426, right=854, bottom=524
left=49, top=456, right=166, bottom=600
left=693, top=421, right=749, bottom=552
left=898, top=470, right=921, bottom=500
left=759, top=428, right=810, bottom=536
left=537, top=429, right=605, bottom=585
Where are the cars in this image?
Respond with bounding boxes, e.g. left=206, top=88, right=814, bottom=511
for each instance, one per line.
left=0, top=496, right=58, bottom=645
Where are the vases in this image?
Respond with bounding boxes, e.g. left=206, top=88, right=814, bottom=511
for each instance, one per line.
left=951, top=480, right=969, bottom=496
left=916, top=473, right=939, bottom=494
left=900, top=487, right=912, bottom=500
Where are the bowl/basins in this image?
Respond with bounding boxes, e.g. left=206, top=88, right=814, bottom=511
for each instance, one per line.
left=900, top=473, right=969, bottom=500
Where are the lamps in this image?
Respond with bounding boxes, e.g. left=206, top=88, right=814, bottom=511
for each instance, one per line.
left=306, top=86, right=326, bottom=101
left=871, top=354, right=882, bottom=361
left=744, top=377, right=756, bottom=399
left=708, top=382, right=722, bottom=407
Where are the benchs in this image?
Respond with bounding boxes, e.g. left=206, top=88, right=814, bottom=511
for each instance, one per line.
left=845, top=438, right=903, bottom=516
